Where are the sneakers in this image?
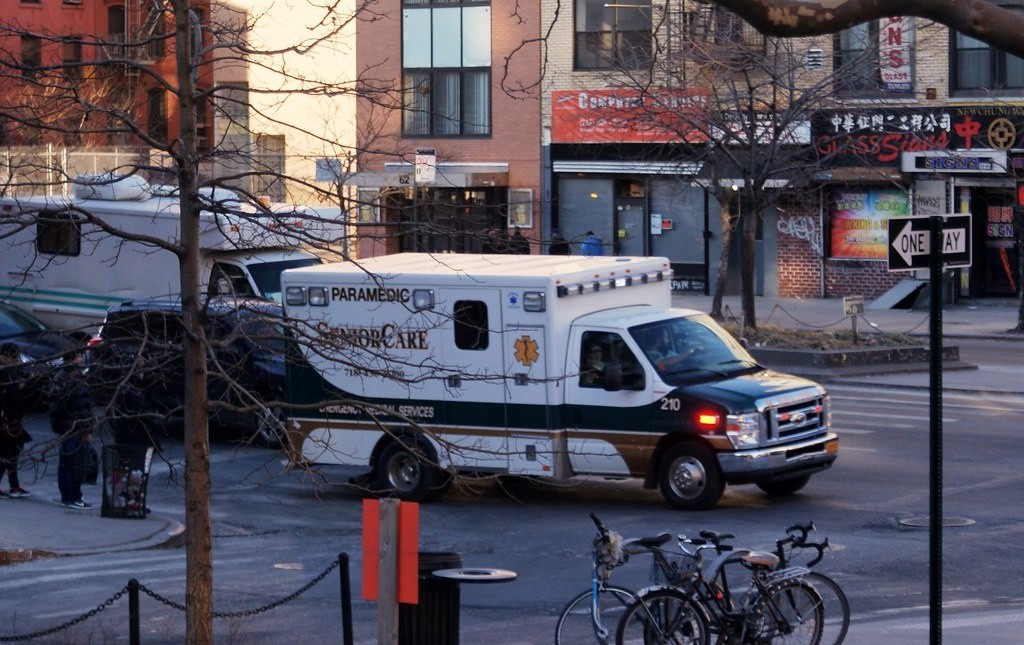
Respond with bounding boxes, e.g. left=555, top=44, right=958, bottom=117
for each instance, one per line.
left=67, top=499, right=92, bottom=510
left=10, top=487, right=30, bottom=498
left=0, top=490, right=9, bottom=499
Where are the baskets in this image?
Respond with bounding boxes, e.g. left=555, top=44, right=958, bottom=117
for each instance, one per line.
left=649, top=547, right=697, bottom=591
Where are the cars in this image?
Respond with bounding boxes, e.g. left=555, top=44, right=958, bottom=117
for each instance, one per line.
left=0, top=300, right=90, bottom=410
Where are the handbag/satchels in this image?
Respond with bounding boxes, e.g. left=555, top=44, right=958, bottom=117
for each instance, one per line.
left=80, top=440, right=100, bottom=485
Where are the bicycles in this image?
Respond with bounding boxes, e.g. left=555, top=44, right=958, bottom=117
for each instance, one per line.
left=555, top=511, right=853, bottom=645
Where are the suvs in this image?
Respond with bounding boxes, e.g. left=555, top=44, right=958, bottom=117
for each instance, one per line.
left=82, top=297, right=318, bottom=450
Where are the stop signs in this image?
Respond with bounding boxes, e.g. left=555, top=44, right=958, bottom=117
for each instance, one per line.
left=886, top=213, right=972, bottom=270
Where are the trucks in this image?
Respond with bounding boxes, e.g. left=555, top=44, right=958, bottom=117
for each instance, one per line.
left=0, top=170, right=348, bottom=357
left=279, top=252, right=838, bottom=515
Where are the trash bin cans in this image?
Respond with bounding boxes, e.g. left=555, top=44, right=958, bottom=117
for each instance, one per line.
left=399, top=548, right=518, bottom=645
left=102, top=443, right=156, bottom=520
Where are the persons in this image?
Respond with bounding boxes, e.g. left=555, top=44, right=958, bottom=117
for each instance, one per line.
left=577, top=341, right=608, bottom=386
left=483, top=224, right=604, bottom=256
left=0, top=342, right=165, bottom=510
left=644, top=328, right=681, bottom=363
